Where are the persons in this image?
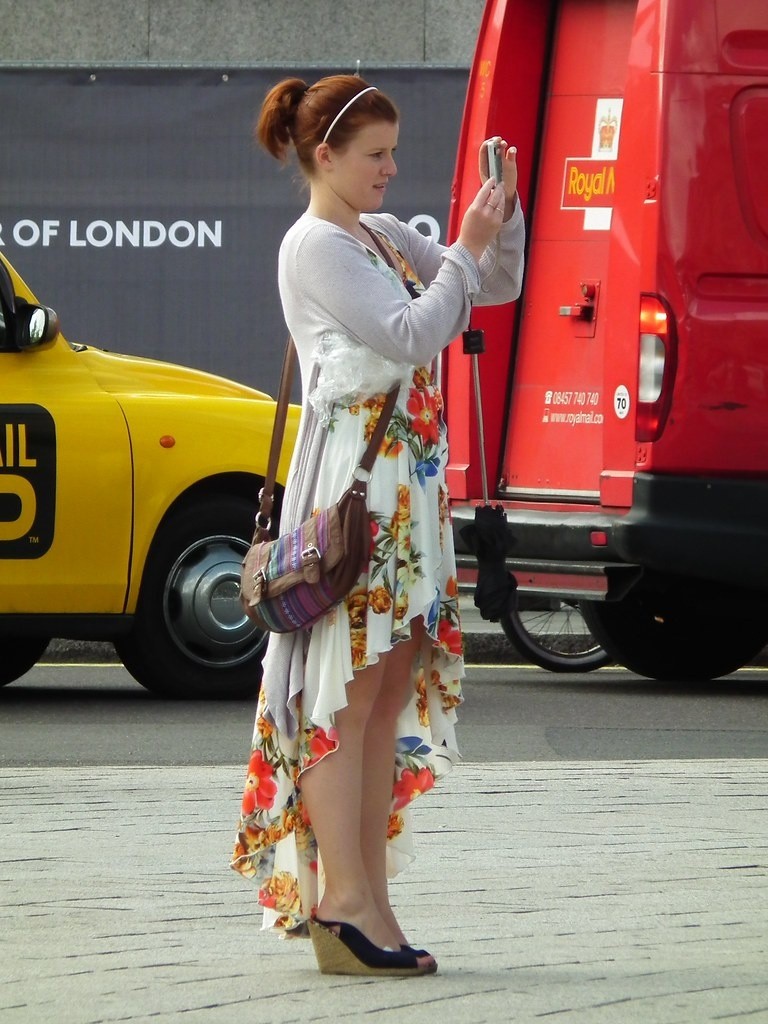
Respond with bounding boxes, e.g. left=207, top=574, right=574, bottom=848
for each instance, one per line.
left=229, top=75, right=526, bottom=976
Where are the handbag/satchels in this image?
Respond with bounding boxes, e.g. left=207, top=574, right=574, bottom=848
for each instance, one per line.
left=241, top=479, right=369, bottom=635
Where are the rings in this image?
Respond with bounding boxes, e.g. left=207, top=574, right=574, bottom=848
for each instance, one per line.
left=487, top=202, right=495, bottom=208
left=501, top=211, right=504, bottom=214
left=496, top=208, right=501, bottom=211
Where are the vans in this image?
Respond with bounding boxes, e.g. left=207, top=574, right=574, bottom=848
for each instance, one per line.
left=426, top=0, right=767, bottom=678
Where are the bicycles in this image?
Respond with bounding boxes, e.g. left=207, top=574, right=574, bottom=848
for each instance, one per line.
left=498, top=595, right=614, bottom=675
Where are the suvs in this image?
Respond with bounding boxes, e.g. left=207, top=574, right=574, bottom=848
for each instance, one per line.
left=1, top=245, right=310, bottom=698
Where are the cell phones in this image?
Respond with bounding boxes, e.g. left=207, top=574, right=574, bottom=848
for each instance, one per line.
left=487, top=141, right=502, bottom=195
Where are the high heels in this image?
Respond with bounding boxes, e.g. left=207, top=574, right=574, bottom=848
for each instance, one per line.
left=307, top=915, right=438, bottom=975
